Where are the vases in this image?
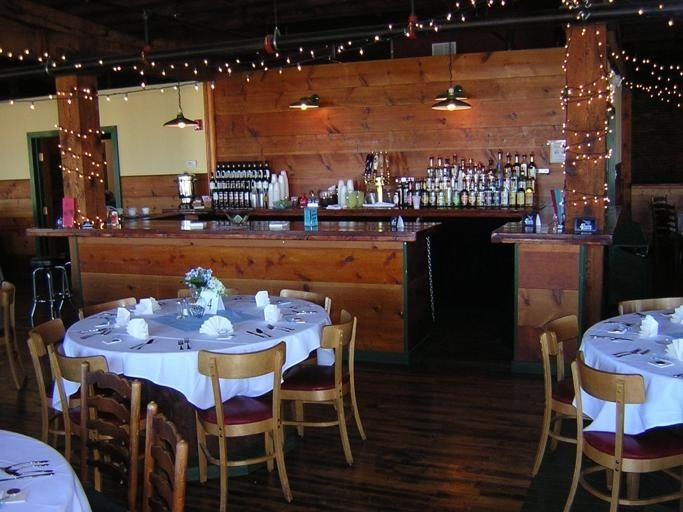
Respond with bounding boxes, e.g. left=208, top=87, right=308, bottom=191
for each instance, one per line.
left=188, top=288, right=206, bottom=318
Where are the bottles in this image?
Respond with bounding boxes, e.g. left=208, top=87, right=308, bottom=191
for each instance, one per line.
left=298, top=193, right=307, bottom=208
left=308, top=193, right=317, bottom=208
left=208, top=161, right=270, bottom=208
left=392, top=149, right=537, bottom=209
left=180, top=299, right=188, bottom=320
left=175, top=300, right=182, bottom=320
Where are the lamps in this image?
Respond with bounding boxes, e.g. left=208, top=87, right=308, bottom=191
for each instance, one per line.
left=432, top=28, right=469, bottom=101
left=433, top=64, right=472, bottom=111
left=288, top=66, right=321, bottom=111
left=161, top=81, right=199, bottom=129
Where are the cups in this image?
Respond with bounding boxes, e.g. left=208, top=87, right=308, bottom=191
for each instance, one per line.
left=128, top=208, right=136, bottom=217
left=336, top=179, right=353, bottom=206
left=266, top=170, right=289, bottom=209
left=142, top=207, right=149, bottom=216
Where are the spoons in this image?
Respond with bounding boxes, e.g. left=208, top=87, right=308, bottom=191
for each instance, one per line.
left=256, top=328, right=271, bottom=337
left=0, top=461, right=54, bottom=476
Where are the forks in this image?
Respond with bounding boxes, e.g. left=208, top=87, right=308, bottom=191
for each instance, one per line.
left=177, top=340, right=183, bottom=350
left=612, top=349, right=641, bottom=356
left=267, top=325, right=295, bottom=333
left=79, top=327, right=111, bottom=339
left=616, top=349, right=648, bottom=358
left=183, top=337, right=190, bottom=349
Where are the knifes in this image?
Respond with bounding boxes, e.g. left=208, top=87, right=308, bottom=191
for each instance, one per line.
left=136, top=339, right=153, bottom=350
left=246, top=331, right=265, bottom=338
left=0, top=473, right=52, bottom=482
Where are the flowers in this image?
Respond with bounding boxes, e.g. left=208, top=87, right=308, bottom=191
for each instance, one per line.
left=181, top=266, right=226, bottom=295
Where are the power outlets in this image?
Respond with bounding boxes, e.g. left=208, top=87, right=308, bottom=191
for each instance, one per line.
left=549, top=139, right=566, bottom=164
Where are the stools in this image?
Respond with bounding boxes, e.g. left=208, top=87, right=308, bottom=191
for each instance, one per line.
left=30, top=257, right=76, bottom=328
